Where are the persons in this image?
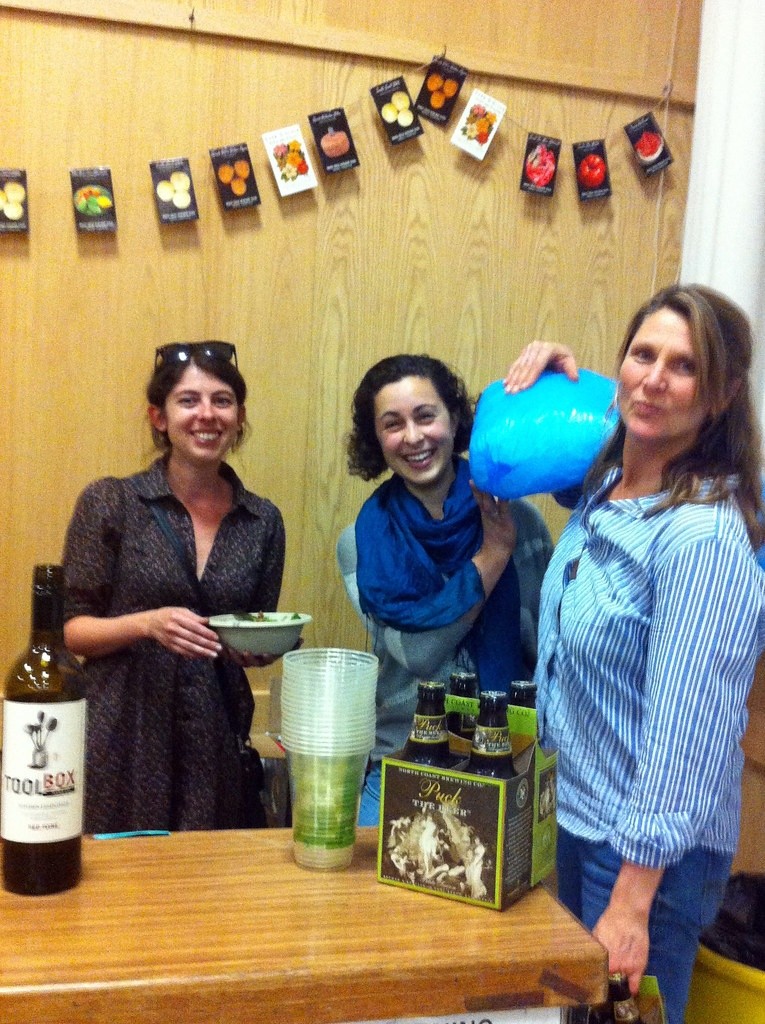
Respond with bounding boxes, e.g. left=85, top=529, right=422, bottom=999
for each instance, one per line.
left=504, top=281, right=765, bottom=1024
left=337, top=354, right=556, bottom=827
left=61, top=341, right=304, bottom=833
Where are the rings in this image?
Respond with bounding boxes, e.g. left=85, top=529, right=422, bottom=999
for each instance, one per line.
left=608, top=973, right=622, bottom=983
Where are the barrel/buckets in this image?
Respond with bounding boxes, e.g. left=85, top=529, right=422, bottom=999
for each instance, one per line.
left=683, top=935, right=765, bottom=1024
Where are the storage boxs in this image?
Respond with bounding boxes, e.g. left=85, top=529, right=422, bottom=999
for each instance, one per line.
left=376, top=694, right=557, bottom=909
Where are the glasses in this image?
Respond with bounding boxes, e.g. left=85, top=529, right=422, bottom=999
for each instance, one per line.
left=154, top=340, right=238, bottom=373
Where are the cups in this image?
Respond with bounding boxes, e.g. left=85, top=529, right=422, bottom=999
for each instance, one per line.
left=280, top=648, right=377, bottom=871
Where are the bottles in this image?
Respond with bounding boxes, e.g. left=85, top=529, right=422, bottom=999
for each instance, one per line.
left=402, top=680, right=450, bottom=775
left=463, top=689, right=520, bottom=781
left=607, top=971, right=643, bottom=1024
left=449, top=672, right=478, bottom=742
left=509, top=680, right=538, bottom=710
left=0, top=564, right=88, bottom=895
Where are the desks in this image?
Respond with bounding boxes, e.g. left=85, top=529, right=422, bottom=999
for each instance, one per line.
left=0, top=824, right=607, bottom=1024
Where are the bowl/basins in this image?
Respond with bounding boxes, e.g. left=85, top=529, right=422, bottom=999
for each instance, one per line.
left=207, top=612, right=313, bottom=659
